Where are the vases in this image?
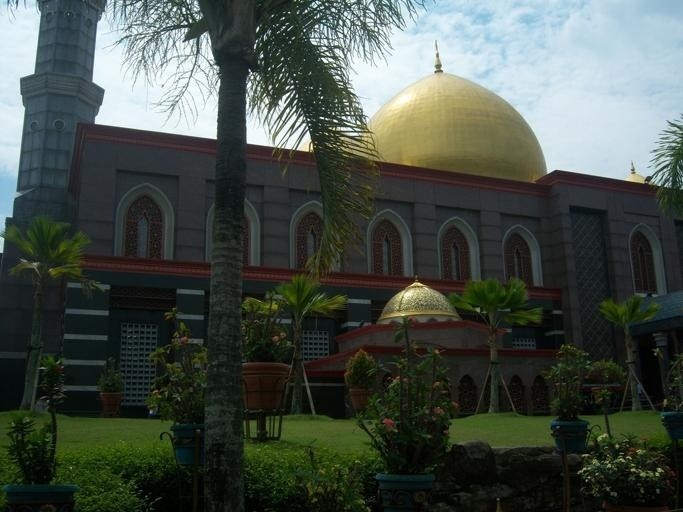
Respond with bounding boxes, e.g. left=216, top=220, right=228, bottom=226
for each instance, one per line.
left=169, top=421, right=209, bottom=466
left=373, top=472, right=436, bottom=512
left=238, top=364, right=289, bottom=409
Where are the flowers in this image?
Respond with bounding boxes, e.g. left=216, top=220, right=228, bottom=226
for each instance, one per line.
left=345, top=339, right=457, bottom=475
left=575, top=433, right=680, bottom=504
left=144, top=307, right=216, bottom=422
left=241, top=312, right=295, bottom=363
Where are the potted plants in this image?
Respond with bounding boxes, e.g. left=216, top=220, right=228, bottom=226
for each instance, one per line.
left=651, top=346, right=683, bottom=439
left=346, top=350, right=390, bottom=411
left=2, top=356, right=79, bottom=512
left=550, top=340, right=627, bottom=454
left=96, top=357, right=125, bottom=417
